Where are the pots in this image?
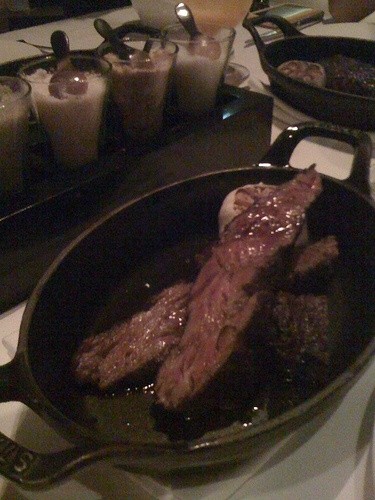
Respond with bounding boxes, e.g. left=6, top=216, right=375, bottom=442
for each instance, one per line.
left=0, top=121, right=375, bottom=489
left=241, top=14, right=375, bottom=132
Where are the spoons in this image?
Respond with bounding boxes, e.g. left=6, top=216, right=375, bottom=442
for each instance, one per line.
left=93, top=17, right=154, bottom=74
left=47, top=30, right=87, bottom=99
left=173, top=2, right=222, bottom=62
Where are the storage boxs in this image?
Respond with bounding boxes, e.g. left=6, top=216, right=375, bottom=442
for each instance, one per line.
left=0, top=84, right=274, bottom=314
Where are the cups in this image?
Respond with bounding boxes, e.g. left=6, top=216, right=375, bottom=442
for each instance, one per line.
left=96, top=37, right=180, bottom=149
left=160, top=21, right=236, bottom=123
left=0, top=74, right=32, bottom=198
left=16, top=53, right=112, bottom=173
